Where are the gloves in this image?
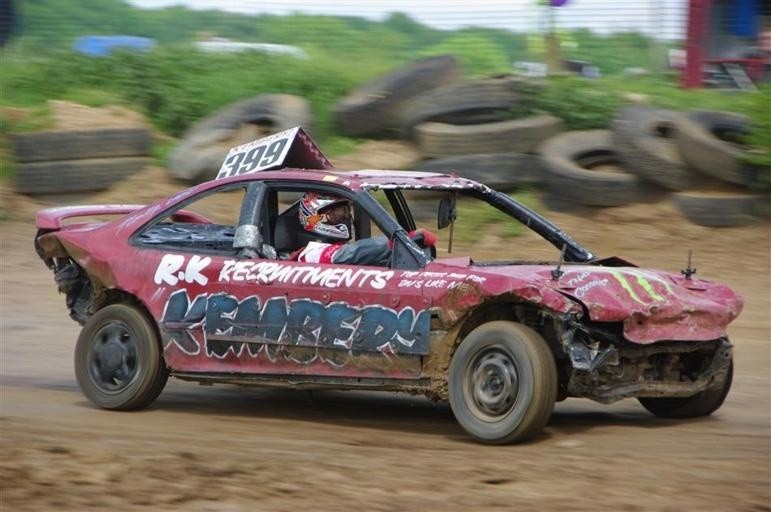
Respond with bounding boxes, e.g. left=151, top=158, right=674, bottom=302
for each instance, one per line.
left=410, top=229, right=437, bottom=248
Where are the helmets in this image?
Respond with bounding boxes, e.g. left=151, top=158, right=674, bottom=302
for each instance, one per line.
left=299, top=192, right=355, bottom=240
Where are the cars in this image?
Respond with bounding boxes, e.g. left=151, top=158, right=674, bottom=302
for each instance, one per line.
left=32, top=130, right=744, bottom=448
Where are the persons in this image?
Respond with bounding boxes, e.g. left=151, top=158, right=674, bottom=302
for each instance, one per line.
left=280, top=191, right=437, bottom=267
